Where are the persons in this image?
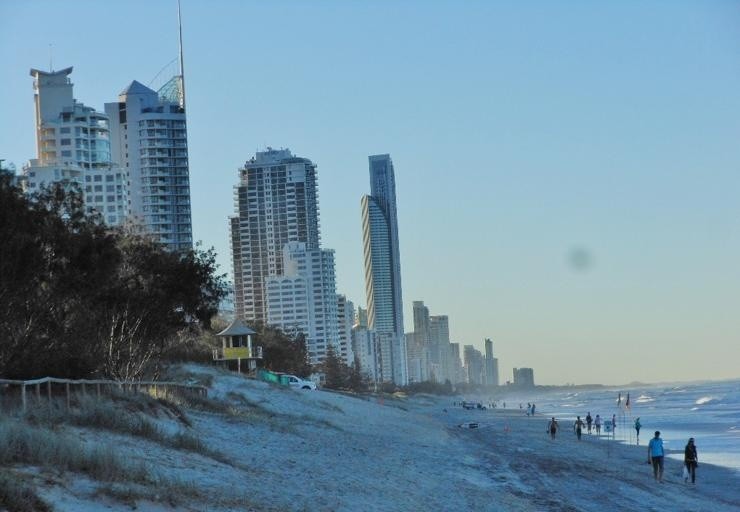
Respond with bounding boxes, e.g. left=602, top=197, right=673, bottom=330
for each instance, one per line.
left=585, top=411, right=617, bottom=436
left=574, top=416, right=584, bottom=440
left=634, top=418, right=643, bottom=442
left=646, top=430, right=664, bottom=484
left=683, top=438, right=699, bottom=485
left=546, top=416, right=559, bottom=438
left=520, top=402, right=536, bottom=417
left=453, top=399, right=508, bottom=409
left=617, top=392, right=630, bottom=408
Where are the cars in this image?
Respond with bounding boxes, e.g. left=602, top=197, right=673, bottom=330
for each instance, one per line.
left=282, top=374, right=316, bottom=390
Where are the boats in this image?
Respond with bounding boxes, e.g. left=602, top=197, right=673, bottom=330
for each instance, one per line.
left=635, top=395, right=656, bottom=405
